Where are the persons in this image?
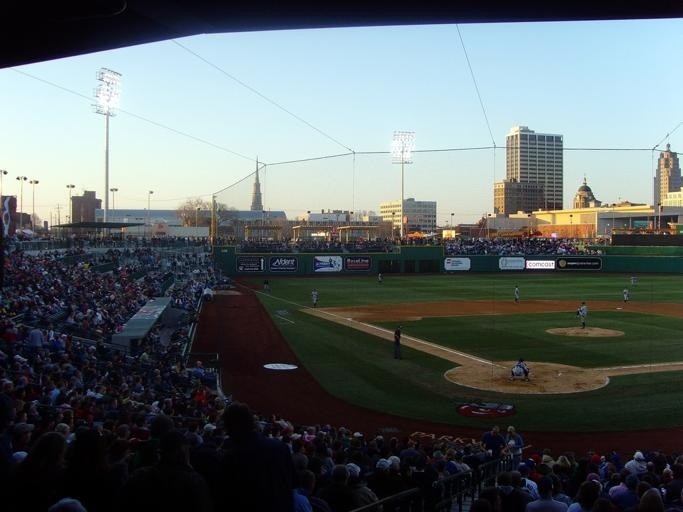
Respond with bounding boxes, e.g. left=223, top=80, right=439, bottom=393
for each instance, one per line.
left=311, top=288, right=319, bottom=307
left=622, top=287, right=629, bottom=304
left=215, top=233, right=603, bottom=257
left=0, top=326, right=682, bottom=511
left=266, top=280, right=268, bottom=289
left=514, top=285, right=520, bottom=303
left=0, top=232, right=216, bottom=327
left=574, top=301, right=587, bottom=328
left=263, top=280, right=266, bottom=289
left=378, top=271, right=383, bottom=283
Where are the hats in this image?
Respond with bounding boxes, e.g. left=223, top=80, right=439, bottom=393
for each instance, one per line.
left=633, top=451, right=645, bottom=460
left=376, top=458, right=393, bottom=471
left=353, top=431, right=364, bottom=437
